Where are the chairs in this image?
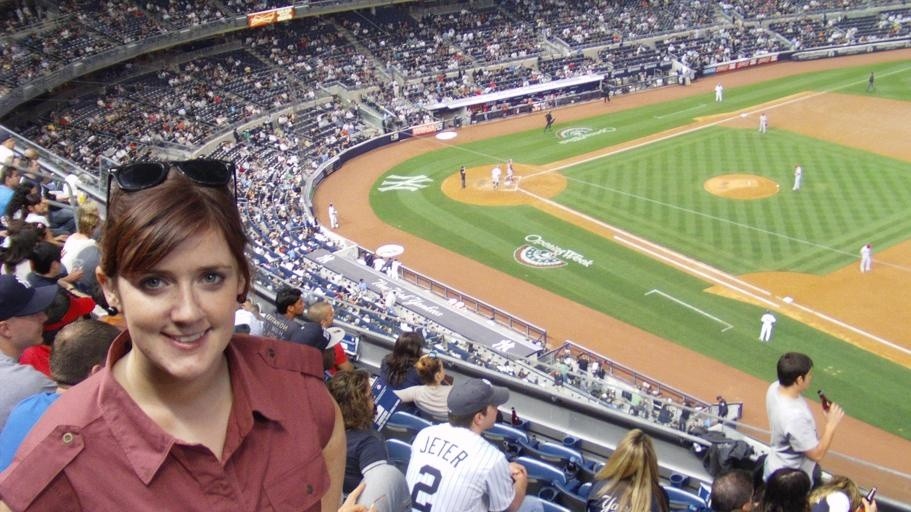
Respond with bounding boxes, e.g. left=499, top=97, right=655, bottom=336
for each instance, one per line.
left=0, top=0, right=911, bottom=512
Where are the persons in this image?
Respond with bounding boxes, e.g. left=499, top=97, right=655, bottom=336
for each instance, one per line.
left=860, top=242, right=873, bottom=270
left=806, top=474, right=879, bottom=512
left=0, top=134, right=404, bottom=511
left=491, top=162, right=503, bottom=187
left=586, top=429, right=669, bottom=512
left=458, top=163, right=466, bottom=189
left=763, top=352, right=845, bottom=496
left=792, top=163, right=802, bottom=192
left=406, top=379, right=546, bottom=512
left=754, top=468, right=811, bottom=512
left=0, top=0, right=911, bottom=134
left=405, top=310, right=729, bottom=433
left=507, top=161, right=513, bottom=186
left=759, top=310, right=776, bottom=341
left=711, top=473, right=755, bottom=512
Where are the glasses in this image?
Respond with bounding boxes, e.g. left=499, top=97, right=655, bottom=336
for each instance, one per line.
left=104, top=160, right=239, bottom=231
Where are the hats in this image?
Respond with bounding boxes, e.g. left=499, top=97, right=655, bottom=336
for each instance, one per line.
left=447, top=378, right=510, bottom=416
left=287, top=321, right=345, bottom=349
left=1, top=275, right=97, bottom=330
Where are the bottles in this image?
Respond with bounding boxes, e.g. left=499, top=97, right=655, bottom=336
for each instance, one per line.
left=567, top=456, right=577, bottom=472
left=854, top=486, right=877, bottom=512
left=510, top=406, right=520, bottom=423
left=817, top=389, right=832, bottom=414
